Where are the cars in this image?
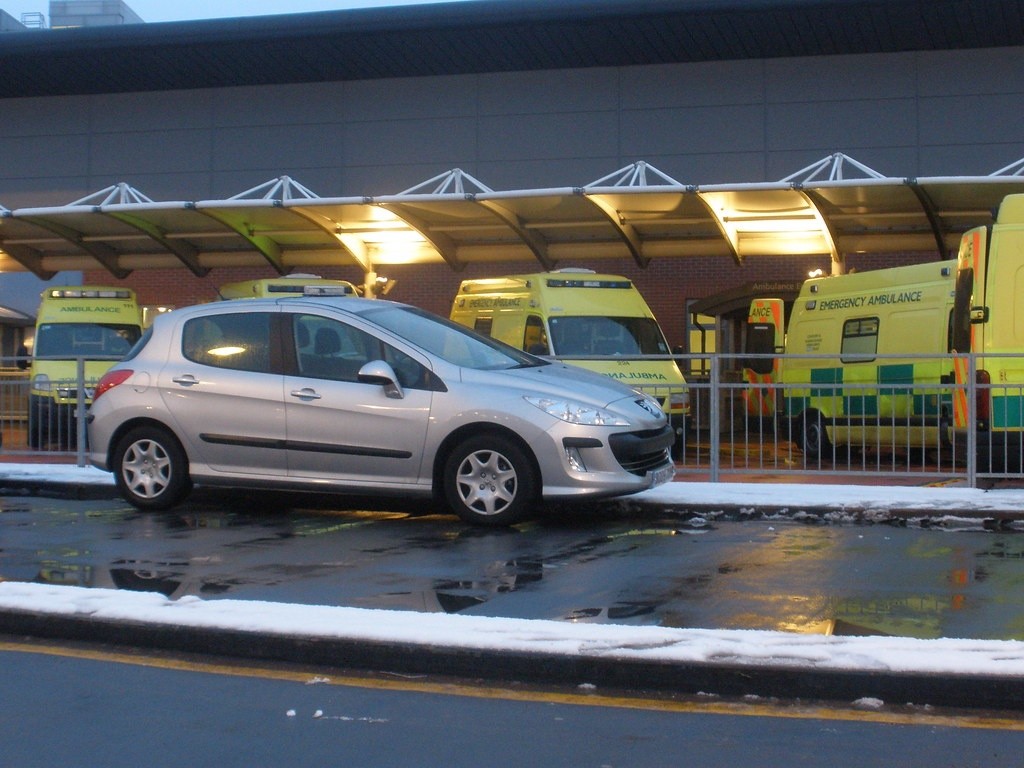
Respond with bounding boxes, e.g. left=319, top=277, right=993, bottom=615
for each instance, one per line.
left=85, top=291, right=677, bottom=523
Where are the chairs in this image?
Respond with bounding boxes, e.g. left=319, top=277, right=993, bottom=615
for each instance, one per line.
left=297, top=322, right=325, bottom=379
left=314, top=327, right=354, bottom=378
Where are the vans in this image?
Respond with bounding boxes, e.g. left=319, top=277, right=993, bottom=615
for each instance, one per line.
left=737, top=257, right=964, bottom=464
left=204, top=272, right=369, bottom=364
left=951, top=194, right=1024, bottom=488
left=441, top=268, right=691, bottom=454
left=26, top=284, right=145, bottom=449
